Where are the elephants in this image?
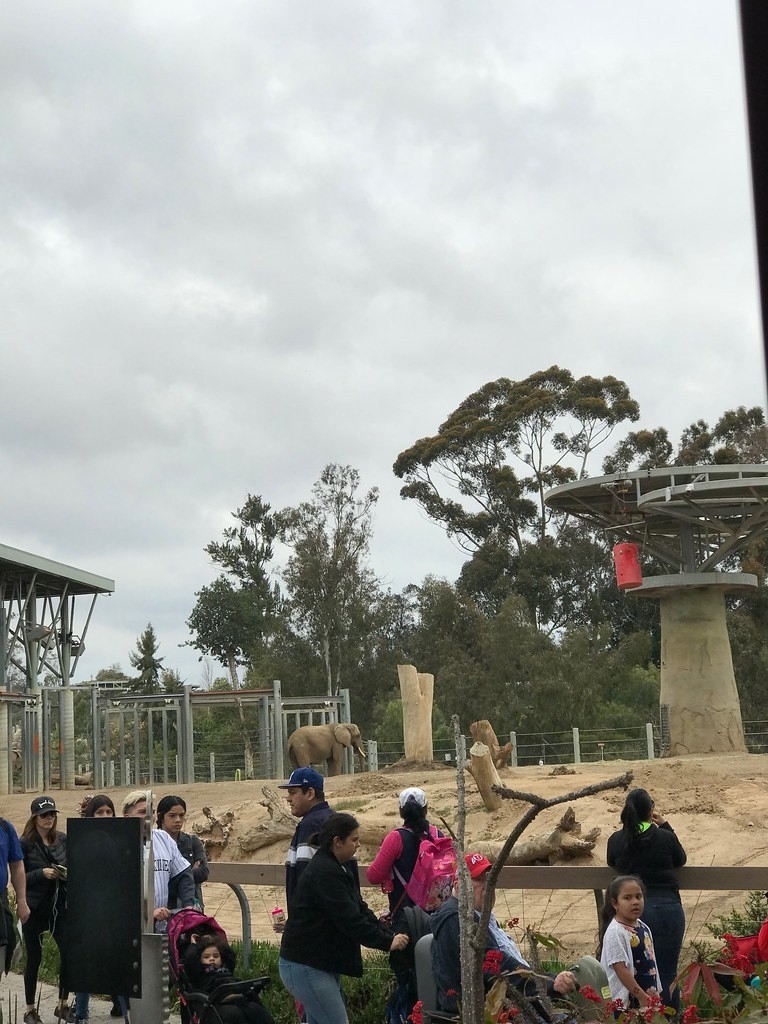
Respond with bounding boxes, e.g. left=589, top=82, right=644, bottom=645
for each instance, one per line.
left=287, top=723, right=366, bottom=777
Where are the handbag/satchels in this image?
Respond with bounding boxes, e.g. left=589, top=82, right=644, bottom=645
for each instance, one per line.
left=379, top=915, right=393, bottom=930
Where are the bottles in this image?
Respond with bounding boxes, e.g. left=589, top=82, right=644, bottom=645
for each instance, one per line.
left=272, top=906, right=286, bottom=932
left=751, top=975, right=766, bottom=990
left=192, top=898, right=202, bottom=912
left=155, top=907, right=186, bottom=930
left=381, top=871, right=394, bottom=894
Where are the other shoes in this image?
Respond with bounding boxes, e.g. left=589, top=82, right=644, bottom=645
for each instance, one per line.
left=69, top=1008, right=89, bottom=1024
left=54, top=1006, right=69, bottom=1020
left=24, top=1008, right=44, bottom=1024
left=110, top=1005, right=123, bottom=1016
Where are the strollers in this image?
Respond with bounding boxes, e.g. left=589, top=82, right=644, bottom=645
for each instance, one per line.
left=155, top=907, right=271, bottom=1024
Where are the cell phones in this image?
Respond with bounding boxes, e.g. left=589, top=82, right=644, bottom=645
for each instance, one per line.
left=57, top=865, right=67, bottom=874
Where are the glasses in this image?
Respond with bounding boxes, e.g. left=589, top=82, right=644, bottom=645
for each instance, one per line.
left=649, top=800, right=655, bottom=811
left=35, top=811, right=56, bottom=819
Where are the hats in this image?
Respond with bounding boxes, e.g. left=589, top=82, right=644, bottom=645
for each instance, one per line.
left=31, top=795, right=60, bottom=815
left=277, top=768, right=323, bottom=789
left=453, top=853, right=492, bottom=884
left=399, top=786, right=427, bottom=809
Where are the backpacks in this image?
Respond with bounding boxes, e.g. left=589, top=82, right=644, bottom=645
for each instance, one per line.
left=390, top=824, right=456, bottom=912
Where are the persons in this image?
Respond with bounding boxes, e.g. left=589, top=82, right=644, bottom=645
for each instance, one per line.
left=0, top=816, right=30, bottom=1024
left=367, top=788, right=444, bottom=1024
left=75, top=791, right=210, bottom=1024
left=273, top=768, right=335, bottom=1022
left=596, top=876, right=663, bottom=1020
left=607, top=788, right=687, bottom=1022
left=279, top=812, right=409, bottom=1024
left=184, top=934, right=274, bottom=1024
left=428, top=854, right=577, bottom=1024
left=18, top=796, right=75, bottom=1024
